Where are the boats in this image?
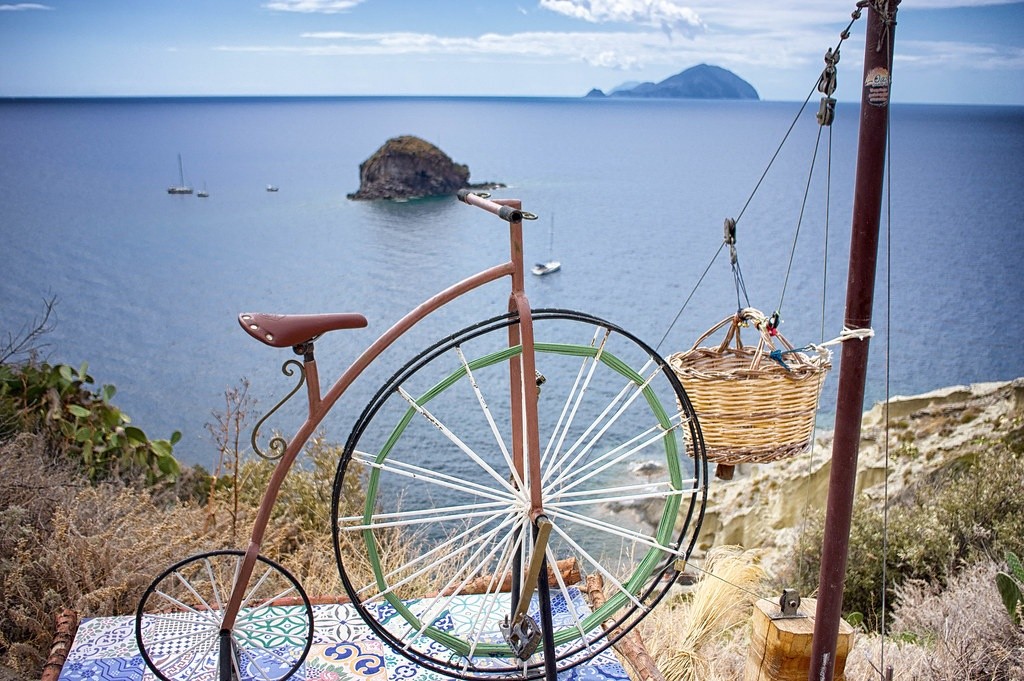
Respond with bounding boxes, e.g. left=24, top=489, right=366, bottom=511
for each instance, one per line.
left=267, top=186, right=279, bottom=190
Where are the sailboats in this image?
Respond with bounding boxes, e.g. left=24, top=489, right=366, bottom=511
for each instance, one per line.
left=198, top=183, right=210, bottom=196
left=167, top=153, right=193, bottom=195
left=530, top=212, right=562, bottom=277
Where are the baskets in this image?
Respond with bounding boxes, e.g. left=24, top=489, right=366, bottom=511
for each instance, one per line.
left=663, top=308, right=834, bottom=465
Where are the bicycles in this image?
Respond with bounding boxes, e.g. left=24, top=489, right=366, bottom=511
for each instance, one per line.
left=135, top=188, right=710, bottom=681
